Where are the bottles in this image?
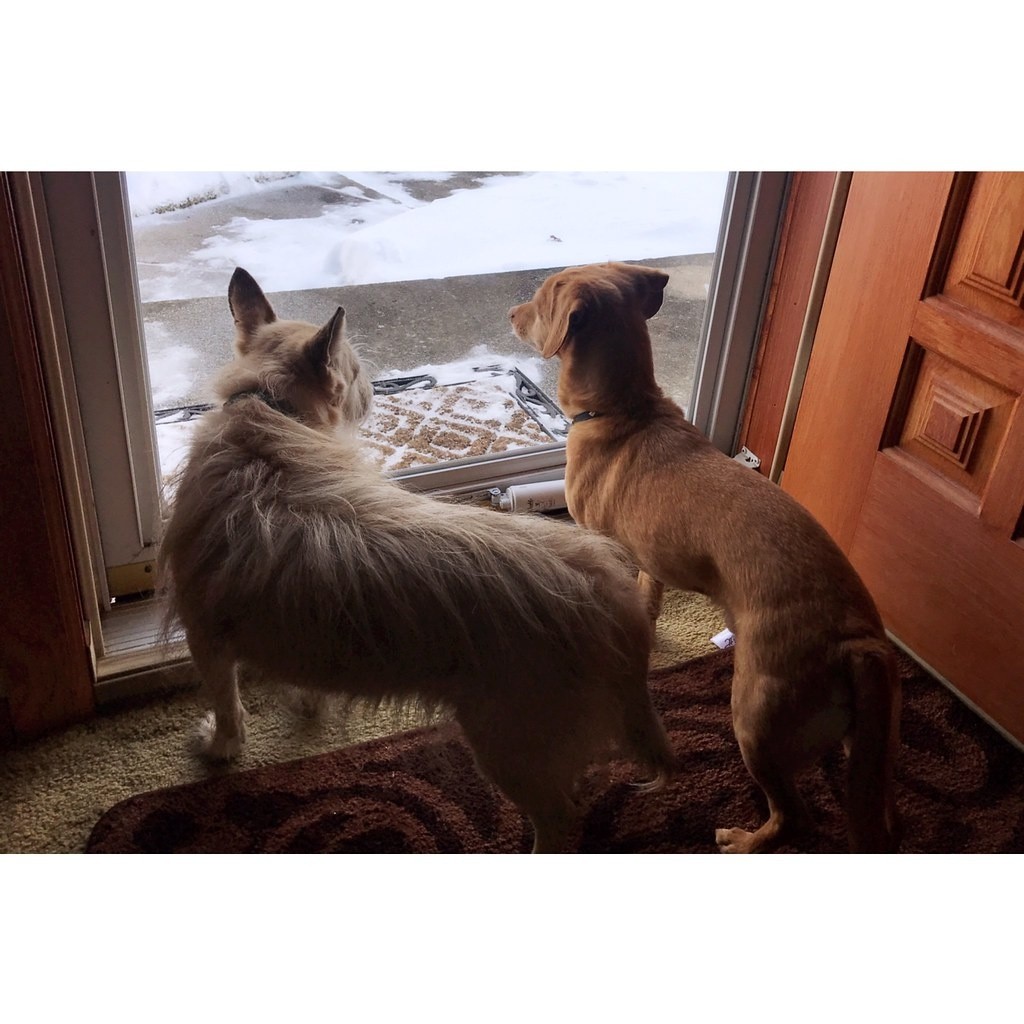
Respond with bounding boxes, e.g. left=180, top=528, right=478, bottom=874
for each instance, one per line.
left=499, top=479, right=567, bottom=514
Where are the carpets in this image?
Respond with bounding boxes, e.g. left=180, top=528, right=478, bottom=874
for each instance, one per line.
left=83, top=643, right=1024, bottom=854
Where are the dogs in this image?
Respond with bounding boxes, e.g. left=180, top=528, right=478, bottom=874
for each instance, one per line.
left=508, top=262, right=900, bottom=854
left=149, top=266, right=681, bottom=854
